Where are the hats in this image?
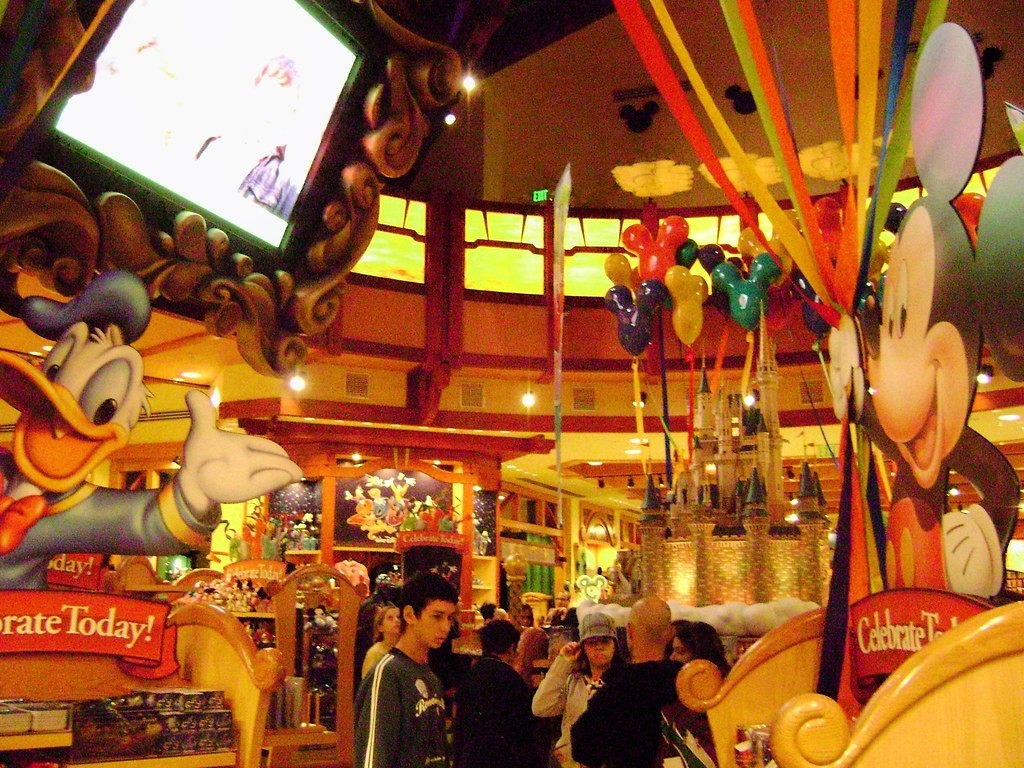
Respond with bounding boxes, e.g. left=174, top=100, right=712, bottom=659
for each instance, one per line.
left=579, top=612, right=620, bottom=649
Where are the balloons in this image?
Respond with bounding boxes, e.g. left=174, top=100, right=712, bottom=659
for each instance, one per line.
left=603, top=196, right=906, bottom=356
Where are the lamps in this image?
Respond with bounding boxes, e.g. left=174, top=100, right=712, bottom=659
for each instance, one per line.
left=782, top=465, right=801, bottom=507
left=976, top=364, right=994, bottom=384
left=597, top=475, right=666, bottom=490
left=744, top=388, right=761, bottom=407
left=949, top=483, right=960, bottom=496
left=632, top=391, right=648, bottom=408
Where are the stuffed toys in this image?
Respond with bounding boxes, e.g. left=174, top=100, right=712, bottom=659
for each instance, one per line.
left=307, top=605, right=333, bottom=627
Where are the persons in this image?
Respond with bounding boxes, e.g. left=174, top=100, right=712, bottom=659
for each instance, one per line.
left=550, top=591, right=578, bottom=626
left=570, top=595, right=720, bottom=768
left=427, top=603, right=733, bottom=768
left=352, top=573, right=460, bottom=768
left=355, top=602, right=402, bottom=701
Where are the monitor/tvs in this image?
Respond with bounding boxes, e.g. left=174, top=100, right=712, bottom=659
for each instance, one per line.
left=49, top=0, right=369, bottom=261
